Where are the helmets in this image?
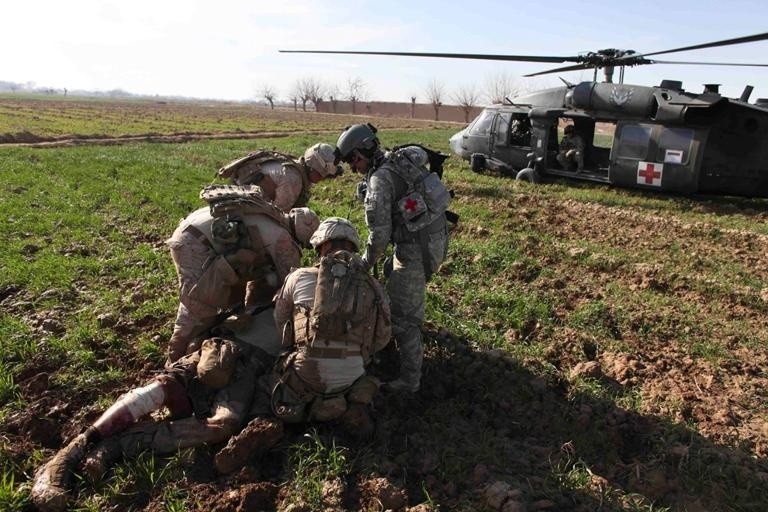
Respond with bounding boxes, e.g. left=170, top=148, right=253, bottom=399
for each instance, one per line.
left=288, top=207, right=361, bottom=250
left=334, top=123, right=380, bottom=170
left=564, top=126, right=575, bottom=135
left=304, top=142, right=343, bottom=181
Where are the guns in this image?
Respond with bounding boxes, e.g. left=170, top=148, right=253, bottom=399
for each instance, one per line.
left=423, top=146, right=459, bottom=230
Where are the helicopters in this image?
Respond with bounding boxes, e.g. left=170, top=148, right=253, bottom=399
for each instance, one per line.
left=277, top=32, right=768, bottom=205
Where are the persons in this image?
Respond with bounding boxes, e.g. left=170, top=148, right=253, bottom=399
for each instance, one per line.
left=228, top=143, right=338, bottom=312
left=556, top=125, right=584, bottom=173
left=213, top=216, right=392, bottom=475
left=31, top=303, right=287, bottom=512
left=336, top=125, right=448, bottom=394
left=164, top=202, right=321, bottom=369
